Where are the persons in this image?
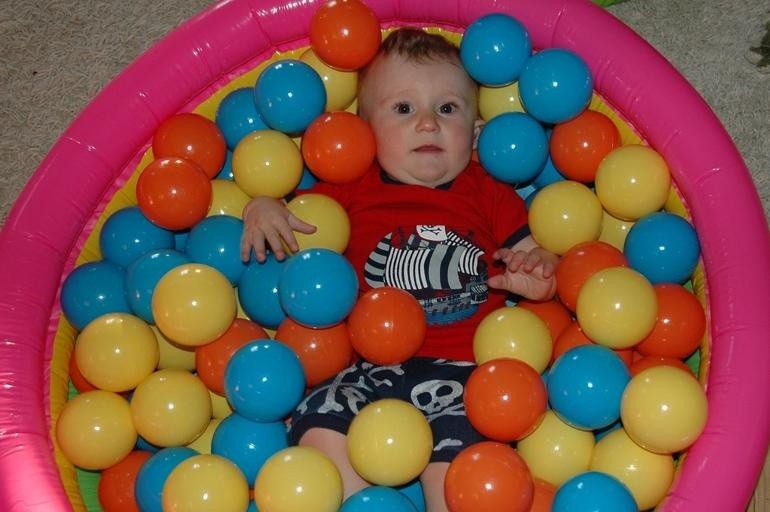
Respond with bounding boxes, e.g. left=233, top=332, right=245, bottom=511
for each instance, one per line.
left=240, top=26, right=556, bottom=512
